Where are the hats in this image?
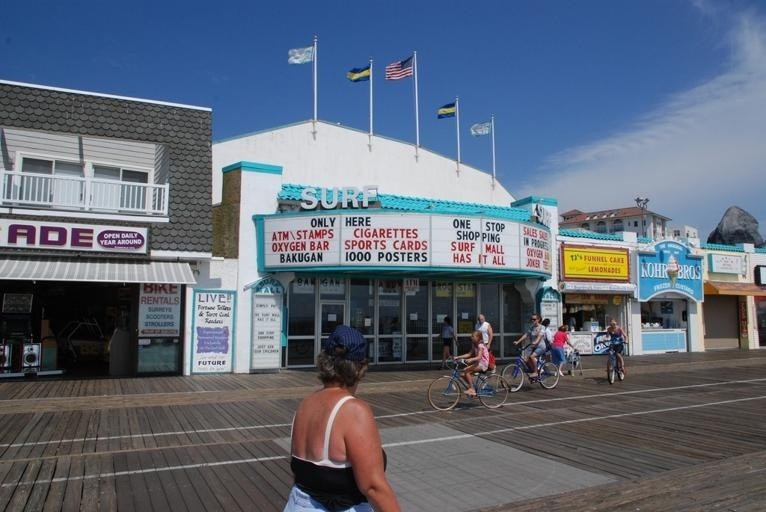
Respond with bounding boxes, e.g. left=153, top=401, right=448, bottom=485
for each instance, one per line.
left=324, top=325, right=367, bottom=361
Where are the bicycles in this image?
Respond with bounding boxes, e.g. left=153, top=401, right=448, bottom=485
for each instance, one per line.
left=597, top=338, right=628, bottom=384
left=500, top=341, right=561, bottom=395
left=427, top=353, right=510, bottom=412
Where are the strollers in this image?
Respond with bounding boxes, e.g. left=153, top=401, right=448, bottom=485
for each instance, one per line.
left=561, top=348, right=584, bottom=378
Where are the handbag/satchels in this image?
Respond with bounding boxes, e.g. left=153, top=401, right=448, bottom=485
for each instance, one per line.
left=545, top=339, right=551, bottom=351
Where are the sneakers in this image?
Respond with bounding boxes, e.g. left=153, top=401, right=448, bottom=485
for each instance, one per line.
left=464, top=388, right=476, bottom=395
left=530, top=373, right=539, bottom=378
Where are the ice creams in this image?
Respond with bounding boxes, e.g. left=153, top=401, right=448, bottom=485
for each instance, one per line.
left=666, top=255, right=679, bottom=286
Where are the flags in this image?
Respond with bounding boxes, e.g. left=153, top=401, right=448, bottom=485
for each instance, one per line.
left=346, top=63, right=370, bottom=82
left=471, top=120, right=492, bottom=136
left=384, top=55, right=415, bottom=80
left=437, top=102, right=456, bottom=119
left=288, top=46, right=314, bottom=64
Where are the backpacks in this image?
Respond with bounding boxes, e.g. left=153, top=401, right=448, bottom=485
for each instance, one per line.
left=478, top=344, right=496, bottom=371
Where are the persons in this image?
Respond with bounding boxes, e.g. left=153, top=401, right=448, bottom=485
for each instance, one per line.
left=454, top=330, right=489, bottom=396
left=440, top=316, right=460, bottom=369
left=606, top=318, right=627, bottom=373
left=283, top=324, right=402, bottom=512
left=514, top=312, right=581, bottom=378
left=475, top=314, right=493, bottom=352
